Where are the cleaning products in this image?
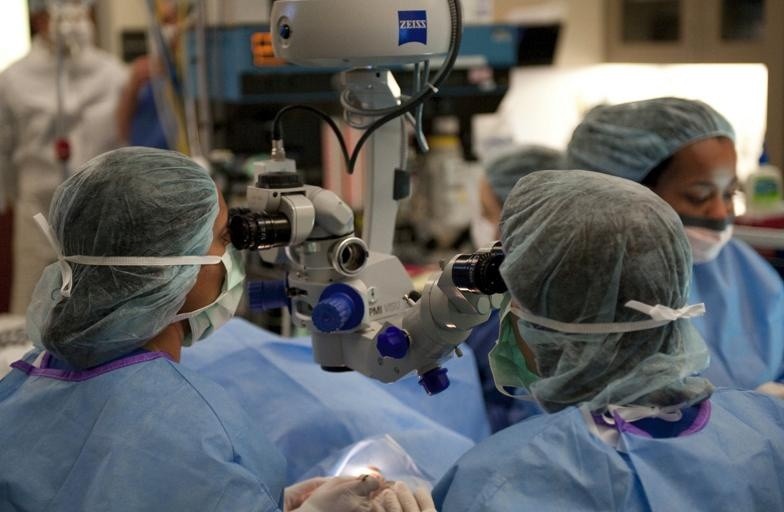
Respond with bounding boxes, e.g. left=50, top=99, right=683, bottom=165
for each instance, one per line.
left=744, top=143, right=784, bottom=216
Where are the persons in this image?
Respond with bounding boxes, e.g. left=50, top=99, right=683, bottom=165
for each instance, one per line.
left=118, top=0, right=191, bottom=150
left=562, top=95, right=784, bottom=398
left=0, top=146, right=393, bottom=512
left=368, top=167, right=784, bottom=512
left=0, top=0, right=135, bottom=316
left=470, top=145, right=567, bottom=253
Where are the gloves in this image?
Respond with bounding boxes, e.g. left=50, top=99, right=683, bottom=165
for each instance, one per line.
left=283, top=474, right=438, bottom=512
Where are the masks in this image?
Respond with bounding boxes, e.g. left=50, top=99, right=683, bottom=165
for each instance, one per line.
left=488, top=292, right=551, bottom=401
left=142, top=243, right=247, bottom=346
left=678, top=214, right=736, bottom=265
